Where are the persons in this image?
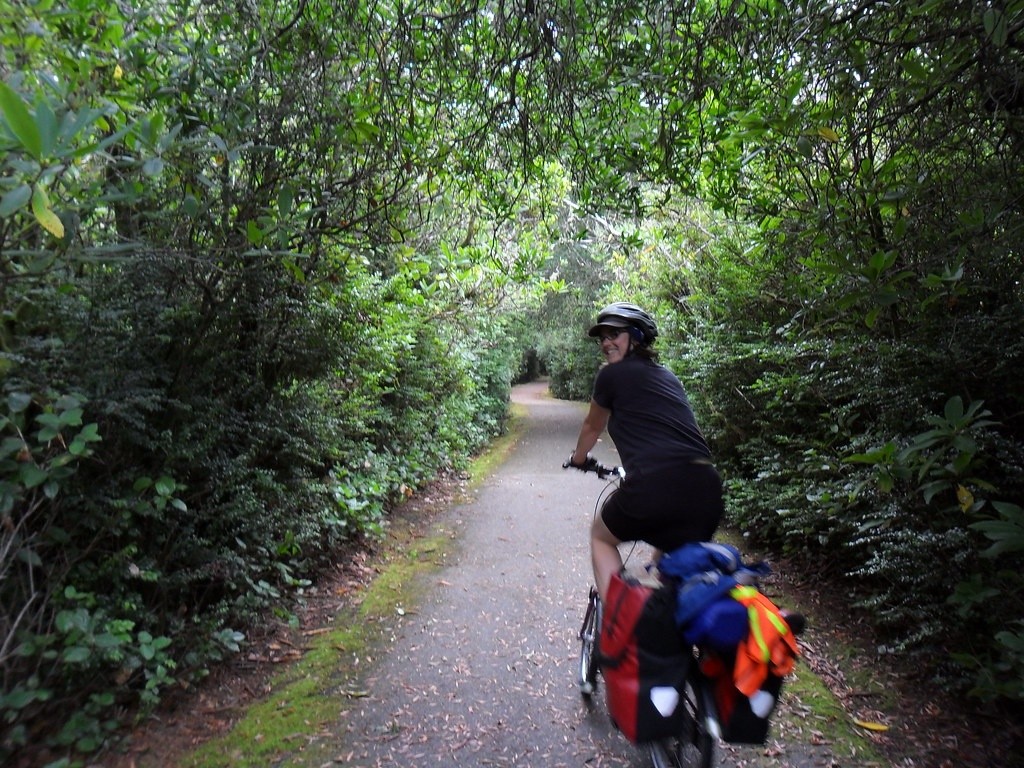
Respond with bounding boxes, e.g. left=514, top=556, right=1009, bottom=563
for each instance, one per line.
left=572, top=302, right=723, bottom=612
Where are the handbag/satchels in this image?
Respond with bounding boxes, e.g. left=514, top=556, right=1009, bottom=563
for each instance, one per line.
left=702, top=605, right=807, bottom=743
left=596, top=565, right=687, bottom=746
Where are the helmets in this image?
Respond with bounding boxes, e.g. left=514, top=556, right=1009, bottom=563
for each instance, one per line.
left=597, top=302, right=658, bottom=341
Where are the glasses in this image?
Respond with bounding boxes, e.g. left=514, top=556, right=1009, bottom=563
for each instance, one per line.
left=594, top=330, right=629, bottom=345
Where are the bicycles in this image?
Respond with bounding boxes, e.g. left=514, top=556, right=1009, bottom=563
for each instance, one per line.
left=561, top=450, right=726, bottom=768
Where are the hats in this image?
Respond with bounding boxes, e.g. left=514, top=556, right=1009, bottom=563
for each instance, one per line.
left=589, top=317, right=634, bottom=337
